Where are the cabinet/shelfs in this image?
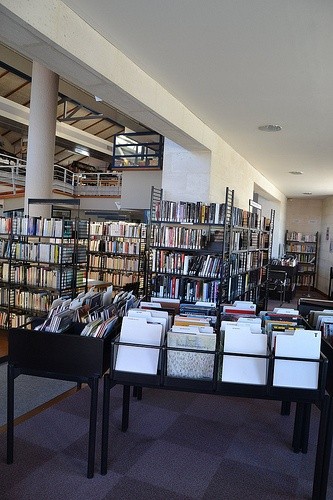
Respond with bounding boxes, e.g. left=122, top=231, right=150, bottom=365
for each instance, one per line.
left=231, top=207, right=275, bottom=311
left=0, top=216, right=91, bottom=330
left=145, top=185, right=235, bottom=307
left=284, top=230, right=320, bottom=288
left=90, top=220, right=150, bottom=302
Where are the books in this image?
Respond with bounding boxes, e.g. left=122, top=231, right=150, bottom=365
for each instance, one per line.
left=0, top=262, right=83, bottom=289
left=287, top=231, right=317, bottom=243
left=151, top=222, right=271, bottom=256
left=0, top=239, right=86, bottom=263
left=154, top=199, right=270, bottom=230
left=287, top=254, right=316, bottom=272
left=0, top=309, right=41, bottom=330
left=88, top=269, right=144, bottom=290
left=89, top=252, right=145, bottom=273
left=0, top=287, right=61, bottom=313
left=90, top=221, right=146, bottom=238
left=115, top=296, right=321, bottom=390
left=89, top=235, right=145, bottom=255
left=0, top=214, right=87, bottom=237
left=297, top=297, right=333, bottom=346
left=151, top=267, right=265, bottom=302
left=285, top=242, right=317, bottom=252
left=42, top=285, right=135, bottom=338
left=151, top=247, right=269, bottom=280
left=294, top=274, right=314, bottom=286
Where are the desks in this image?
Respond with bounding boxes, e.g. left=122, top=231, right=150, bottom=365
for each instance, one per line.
left=268, top=264, right=298, bottom=304
